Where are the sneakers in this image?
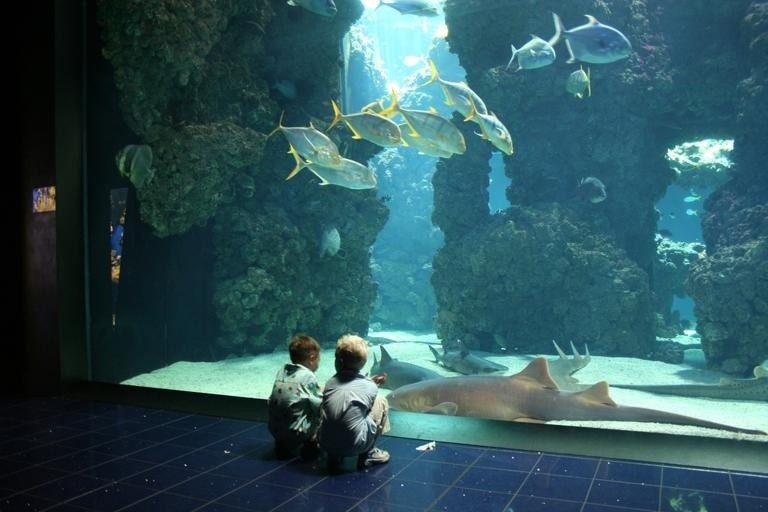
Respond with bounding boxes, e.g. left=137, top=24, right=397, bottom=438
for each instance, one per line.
left=357, top=448, right=390, bottom=467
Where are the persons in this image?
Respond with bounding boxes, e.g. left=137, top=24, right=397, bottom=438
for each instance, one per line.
left=316, top=333, right=396, bottom=467
left=265, top=334, right=327, bottom=459
